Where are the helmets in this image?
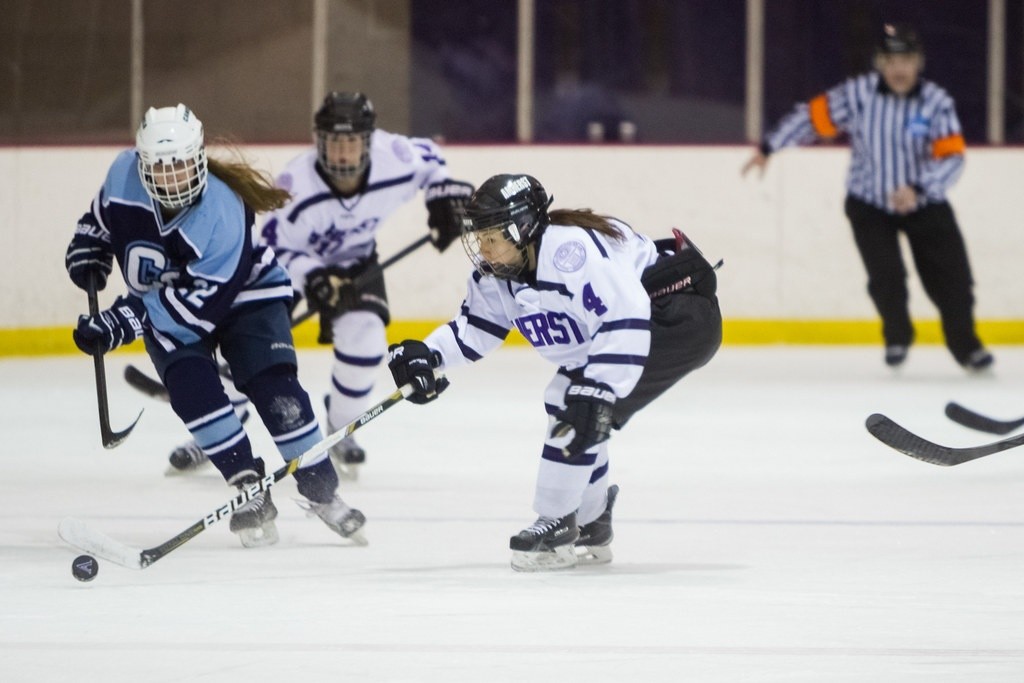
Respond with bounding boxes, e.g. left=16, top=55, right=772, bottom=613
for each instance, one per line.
left=314, top=91, right=376, bottom=132
left=463, top=173, right=551, bottom=250
left=136, top=103, right=204, bottom=164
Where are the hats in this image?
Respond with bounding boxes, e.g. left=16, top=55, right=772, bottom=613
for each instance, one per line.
left=874, top=17, right=927, bottom=52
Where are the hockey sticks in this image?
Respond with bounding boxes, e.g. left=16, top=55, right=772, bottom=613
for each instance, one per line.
left=945, top=401, right=1024, bottom=437
left=86, top=272, right=145, bottom=451
left=123, top=232, right=435, bottom=405
left=864, top=412, right=1024, bottom=469
left=57, top=369, right=440, bottom=571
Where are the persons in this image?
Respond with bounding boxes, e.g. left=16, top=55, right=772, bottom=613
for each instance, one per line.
left=387, top=173, right=726, bottom=573
left=65, top=102, right=371, bottom=550
left=165, top=91, right=476, bottom=478
left=739, top=18, right=995, bottom=372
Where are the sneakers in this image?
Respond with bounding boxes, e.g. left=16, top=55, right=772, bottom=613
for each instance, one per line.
left=967, top=348, right=995, bottom=369
left=571, top=484, right=619, bottom=565
left=165, top=438, right=210, bottom=476
left=229, top=456, right=279, bottom=548
left=323, top=395, right=366, bottom=481
left=509, top=508, right=580, bottom=571
left=294, top=483, right=370, bottom=546
left=884, top=326, right=917, bottom=367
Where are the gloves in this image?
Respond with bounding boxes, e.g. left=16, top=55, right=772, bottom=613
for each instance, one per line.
left=303, top=264, right=364, bottom=318
left=72, top=295, right=149, bottom=355
left=424, top=180, right=475, bottom=253
left=65, top=212, right=114, bottom=291
left=549, top=377, right=617, bottom=462
left=388, top=339, right=451, bottom=405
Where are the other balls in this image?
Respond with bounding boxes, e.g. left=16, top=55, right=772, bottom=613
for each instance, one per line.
left=71, top=555, right=98, bottom=582
